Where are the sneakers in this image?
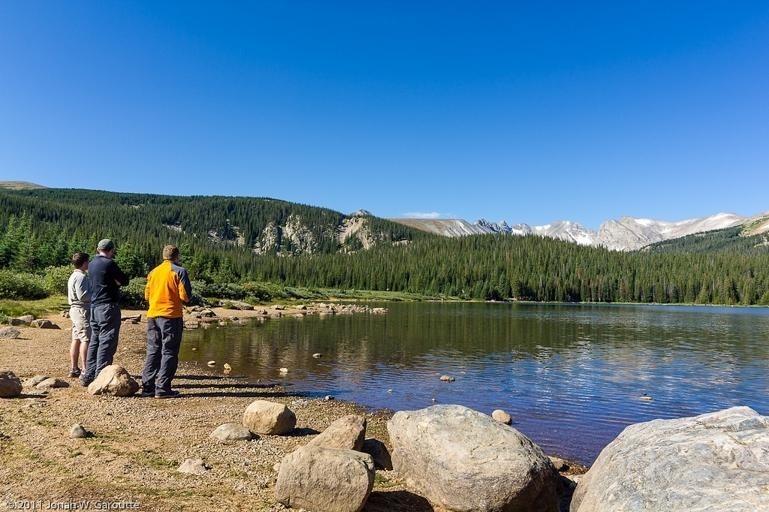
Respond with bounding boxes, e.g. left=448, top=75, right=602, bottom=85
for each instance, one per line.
left=142, top=385, right=180, bottom=399
left=70, top=367, right=86, bottom=378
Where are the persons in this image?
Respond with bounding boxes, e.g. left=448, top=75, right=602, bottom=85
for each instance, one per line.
left=78, top=239, right=129, bottom=387
left=67, top=251, right=92, bottom=378
left=140, top=244, right=192, bottom=398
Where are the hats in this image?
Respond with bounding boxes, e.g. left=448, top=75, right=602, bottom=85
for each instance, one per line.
left=97, top=238, right=115, bottom=250
left=162, top=244, right=180, bottom=260
